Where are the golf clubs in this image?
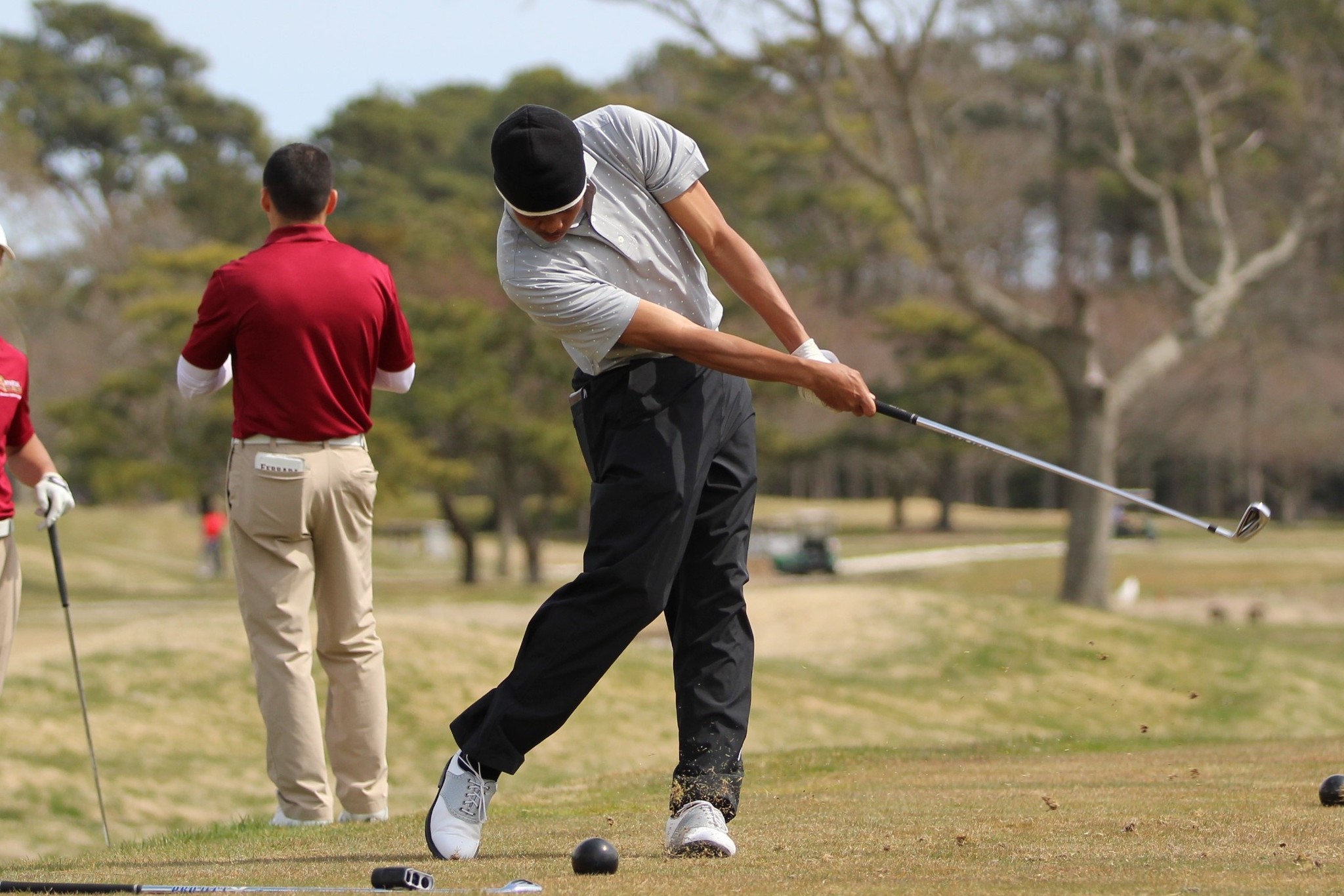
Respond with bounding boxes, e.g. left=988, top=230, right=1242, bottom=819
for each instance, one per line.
left=874, top=398, right=1269, bottom=542
left=45, top=521, right=111, bottom=852
left=0, top=879, right=544, bottom=895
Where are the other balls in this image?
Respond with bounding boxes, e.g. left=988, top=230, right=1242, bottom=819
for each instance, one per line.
left=1318, top=774, right=1344, bottom=807
left=571, top=836, right=621, bottom=875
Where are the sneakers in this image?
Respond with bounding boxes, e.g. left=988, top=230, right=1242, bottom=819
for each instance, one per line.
left=425, top=747, right=498, bottom=859
left=267, top=806, right=332, bottom=824
left=338, top=809, right=389, bottom=823
left=665, top=800, right=736, bottom=856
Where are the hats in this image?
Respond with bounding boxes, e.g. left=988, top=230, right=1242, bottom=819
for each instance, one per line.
left=491, top=104, right=586, bottom=217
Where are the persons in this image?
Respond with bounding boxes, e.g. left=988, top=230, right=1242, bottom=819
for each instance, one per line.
left=422, top=105, right=879, bottom=858
left=0, top=222, right=77, bottom=729
left=175, top=140, right=415, bottom=824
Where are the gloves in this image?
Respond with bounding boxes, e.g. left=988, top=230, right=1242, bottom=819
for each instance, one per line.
left=34, top=472, right=76, bottom=527
